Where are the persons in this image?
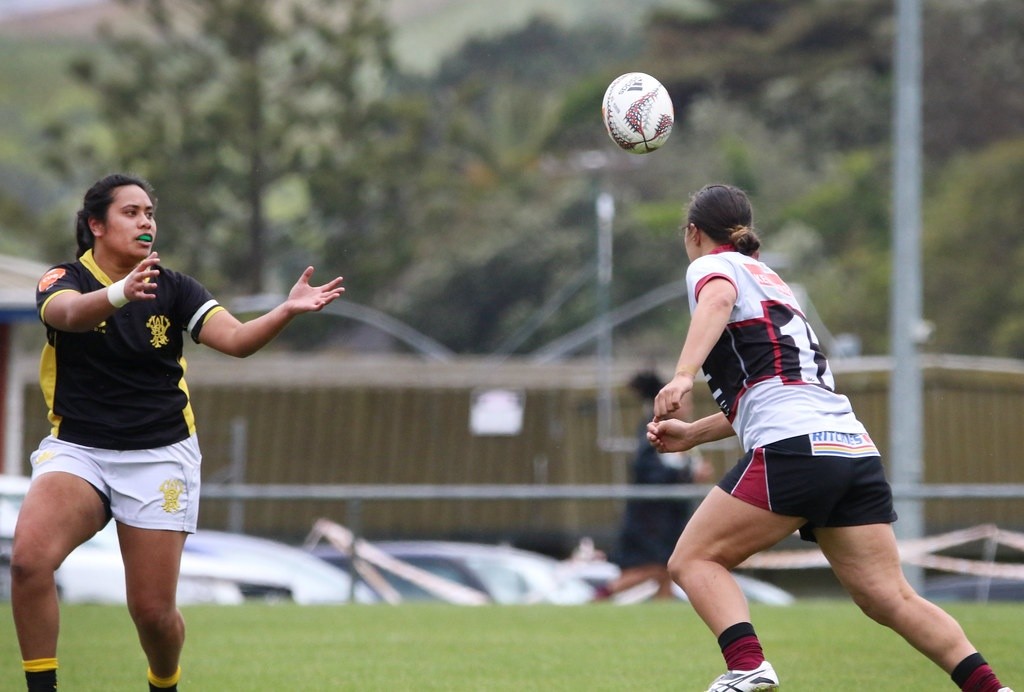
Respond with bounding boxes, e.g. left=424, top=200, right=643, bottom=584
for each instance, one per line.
left=619, top=370, right=708, bottom=562
left=646, top=183, right=1012, bottom=692
left=10, top=174, right=345, bottom=692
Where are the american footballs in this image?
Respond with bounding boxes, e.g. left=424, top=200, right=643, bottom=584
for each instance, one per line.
left=600, top=71, right=675, bottom=155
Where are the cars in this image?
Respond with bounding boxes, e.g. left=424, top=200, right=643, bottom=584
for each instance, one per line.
left=0, top=468, right=797, bottom=608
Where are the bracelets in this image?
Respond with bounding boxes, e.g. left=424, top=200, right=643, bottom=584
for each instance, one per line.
left=106, top=279, right=130, bottom=309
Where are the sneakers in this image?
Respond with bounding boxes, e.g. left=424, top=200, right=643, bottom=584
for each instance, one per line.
left=706, top=660, right=780, bottom=692
